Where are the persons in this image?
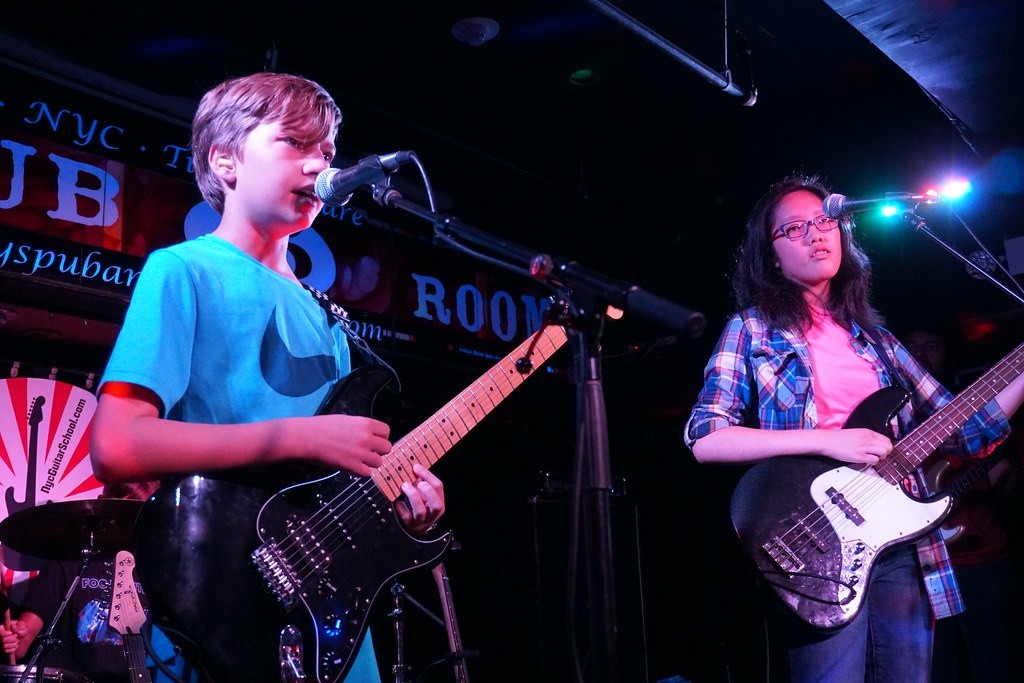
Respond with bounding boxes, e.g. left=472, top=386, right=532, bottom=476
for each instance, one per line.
left=91, top=73, right=446, bottom=683
left=681, top=175, right=1024, bottom=683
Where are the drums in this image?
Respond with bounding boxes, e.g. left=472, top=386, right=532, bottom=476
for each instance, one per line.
left=1, top=660, right=100, bottom=683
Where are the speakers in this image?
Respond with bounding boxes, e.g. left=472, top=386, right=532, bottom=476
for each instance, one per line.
left=462, top=497, right=655, bottom=683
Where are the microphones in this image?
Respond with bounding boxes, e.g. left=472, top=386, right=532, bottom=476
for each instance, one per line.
left=313, top=149, right=417, bottom=208
left=820, top=192, right=930, bottom=219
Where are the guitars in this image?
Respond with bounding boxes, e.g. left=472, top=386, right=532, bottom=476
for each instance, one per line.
left=729, top=344, right=1024, bottom=630
left=139, top=321, right=571, bottom=683
left=108, top=548, right=151, bottom=683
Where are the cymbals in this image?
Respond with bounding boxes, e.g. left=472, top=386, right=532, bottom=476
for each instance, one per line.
left=0, top=498, right=146, bottom=559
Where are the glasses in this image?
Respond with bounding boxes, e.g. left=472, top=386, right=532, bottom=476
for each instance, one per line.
left=771, top=214, right=839, bottom=239
